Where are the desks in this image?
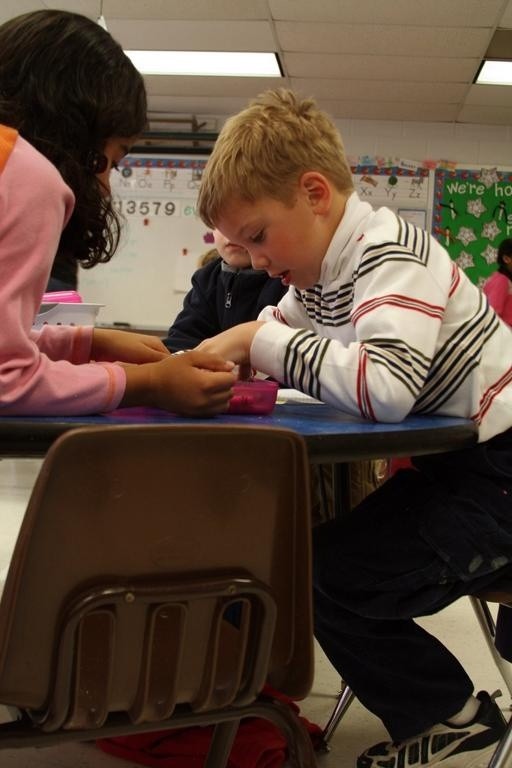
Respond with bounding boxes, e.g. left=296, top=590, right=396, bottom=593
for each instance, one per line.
left=0, top=401, right=481, bottom=763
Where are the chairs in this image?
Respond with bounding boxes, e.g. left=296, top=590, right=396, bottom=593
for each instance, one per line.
left=0, top=418, right=323, bottom=763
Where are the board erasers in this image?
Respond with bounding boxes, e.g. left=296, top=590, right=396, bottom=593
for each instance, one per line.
left=114, top=323, right=129, bottom=328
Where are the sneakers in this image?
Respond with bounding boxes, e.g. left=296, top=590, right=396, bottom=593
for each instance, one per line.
left=354, top=685, right=508, bottom=768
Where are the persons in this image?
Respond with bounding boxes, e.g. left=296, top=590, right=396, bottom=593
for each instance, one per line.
left=0, top=8, right=245, bottom=423
left=161, top=223, right=286, bottom=355
left=191, top=84, right=512, bottom=768
left=479, top=236, right=512, bottom=330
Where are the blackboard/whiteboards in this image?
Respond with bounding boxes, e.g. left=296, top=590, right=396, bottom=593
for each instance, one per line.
left=77, top=153, right=435, bottom=336
